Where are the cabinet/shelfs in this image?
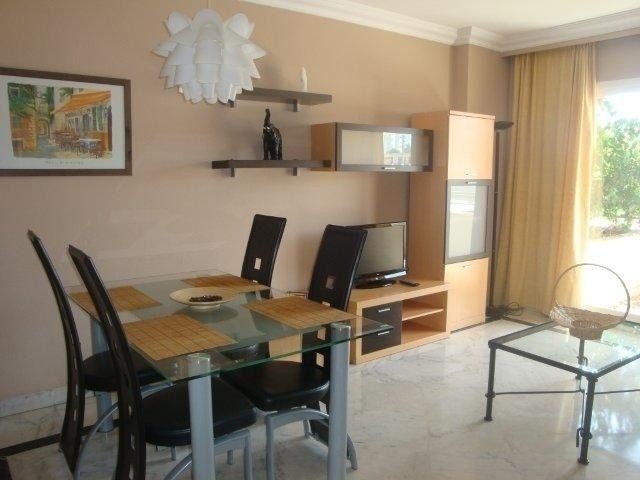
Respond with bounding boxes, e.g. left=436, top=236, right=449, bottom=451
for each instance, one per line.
left=308, top=118, right=435, bottom=173
left=211, top=77, right=336, bottom=174
left=348, top=278, right=450, bottom=361
left=411, top=111, right=492, bottom=331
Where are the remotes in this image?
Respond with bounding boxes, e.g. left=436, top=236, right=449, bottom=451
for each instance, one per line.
left=400, top=279, right=421, bottom=287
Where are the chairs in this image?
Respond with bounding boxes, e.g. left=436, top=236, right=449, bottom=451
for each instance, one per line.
left=27, top=228, right=175, bottom=480
left=68, top=245, right=256, bottom=480
left=208, top=214, right=358, bottom=476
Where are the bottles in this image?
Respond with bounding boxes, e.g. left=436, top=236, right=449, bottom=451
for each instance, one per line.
left=298, top=66, right=308, bottom=93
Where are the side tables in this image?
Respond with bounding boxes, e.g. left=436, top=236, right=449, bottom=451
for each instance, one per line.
left=485, top=319, right=640, bottom=467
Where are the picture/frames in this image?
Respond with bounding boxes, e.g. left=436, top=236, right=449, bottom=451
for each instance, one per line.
left=0, top=66, right=136, bottom=178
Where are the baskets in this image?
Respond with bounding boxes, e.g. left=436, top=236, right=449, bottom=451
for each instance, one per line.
left=548, top=262, right=631, bottom=340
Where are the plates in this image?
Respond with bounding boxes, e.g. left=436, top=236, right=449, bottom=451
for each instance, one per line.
left=168, top=287, right=236, bottom=309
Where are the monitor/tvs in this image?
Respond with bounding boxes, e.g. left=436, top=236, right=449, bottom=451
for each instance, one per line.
left=345, top=221, right=409, bottom=289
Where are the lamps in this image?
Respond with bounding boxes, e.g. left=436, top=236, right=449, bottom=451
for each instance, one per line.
left=155, top=0, right=256, bottom=106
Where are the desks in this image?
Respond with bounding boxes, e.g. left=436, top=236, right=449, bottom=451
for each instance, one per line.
left=56, top=265, right=393, bottom=480
